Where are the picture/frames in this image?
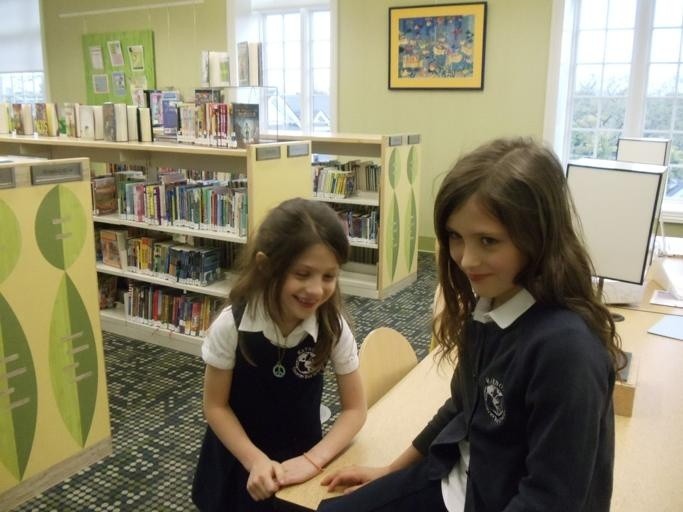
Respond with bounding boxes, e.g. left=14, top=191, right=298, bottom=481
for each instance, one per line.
left=386, top=2, right=487, bottom=92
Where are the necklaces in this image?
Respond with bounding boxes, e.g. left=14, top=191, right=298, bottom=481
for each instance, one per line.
left=270, top=317, right=288, bottom=378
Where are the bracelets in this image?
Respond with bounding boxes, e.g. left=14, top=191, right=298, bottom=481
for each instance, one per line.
left=303, top=451, right=323, bottom=473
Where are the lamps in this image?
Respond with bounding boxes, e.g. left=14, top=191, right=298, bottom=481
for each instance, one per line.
left=559, top=137, right=674, bottom=419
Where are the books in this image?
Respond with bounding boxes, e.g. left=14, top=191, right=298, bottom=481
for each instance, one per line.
left=1, top=89, right=260, bottom=150
left=201, top=50, right=230, bottom=86
left=94, top=223, right=246, bottom=289
left=349, top=246, right=380, bottom=290
left=96, top=271, right=230, bottom=338
left=326, top=202, right=380, bottom=241
left=90, top=162, right=248, bottom=237
left=313, top=159, right=381, bottom=199
left=237, top=40, right=259, bottom=85
left=256, top=41, right=264, bottom=86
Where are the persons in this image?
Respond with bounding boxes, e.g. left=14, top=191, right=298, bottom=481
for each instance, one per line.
left=313, top=135, right=630, bottom=512
left=190, top=195, right=369, bottom=511
